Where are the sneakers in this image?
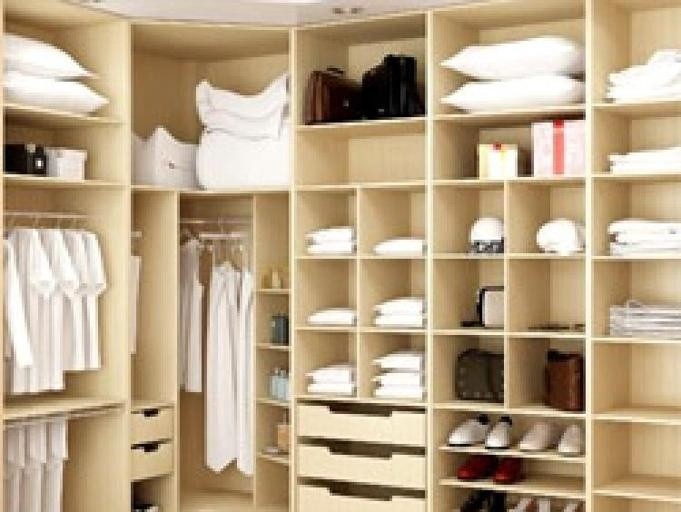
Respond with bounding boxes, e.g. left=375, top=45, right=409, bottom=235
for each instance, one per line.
left=485, top=417, right=513, bottom=449
left=447, top=415, right=491, bottom=445
left=518, top=422, right=557, bottom=451
left=558, top=423, right=584, bottom=454
left=494, top=458, right=526, bottom=483
left=457, top=455, right=498, bottom=479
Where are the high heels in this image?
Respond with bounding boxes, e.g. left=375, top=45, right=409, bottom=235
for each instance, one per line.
left=460, top=491, right=581, bottom=512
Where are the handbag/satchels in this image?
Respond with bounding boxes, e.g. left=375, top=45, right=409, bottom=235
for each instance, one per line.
left=455, top=348, right=503, bottom=401
left=362, top=54, right=418, bottom=119
left=546, top=350, right=581, bottom=410
left=307, top=65, right=361, bottom=129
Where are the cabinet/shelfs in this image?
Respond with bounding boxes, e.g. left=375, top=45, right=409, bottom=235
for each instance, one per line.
left=2, top=1, right=681, bottom=511
